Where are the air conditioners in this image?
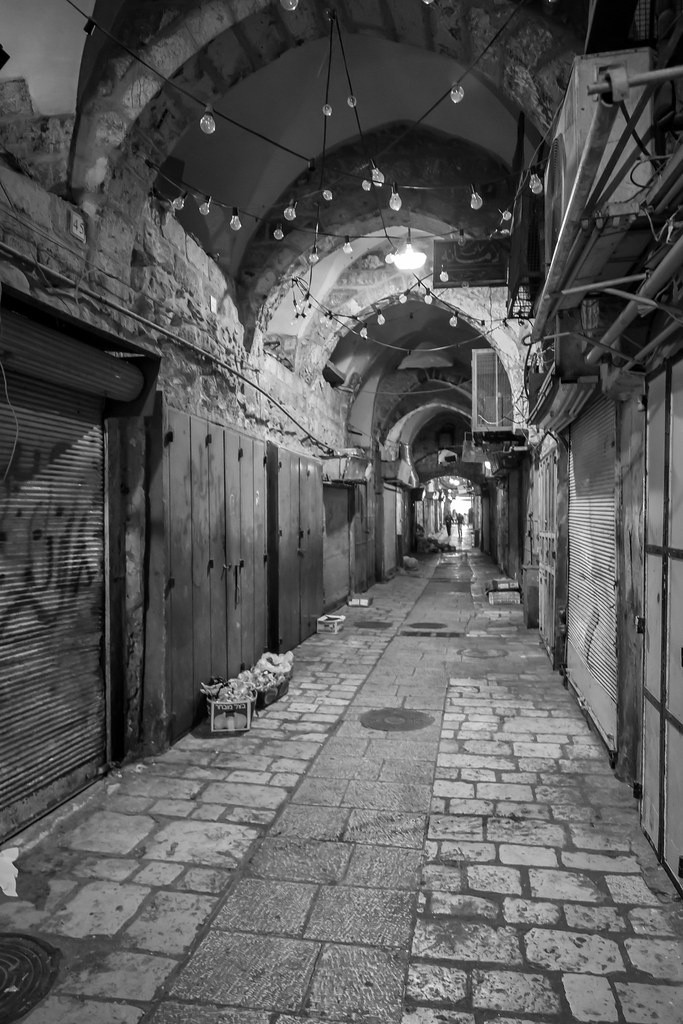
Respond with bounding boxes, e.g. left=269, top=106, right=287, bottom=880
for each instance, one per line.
left=545, top=47, right=655, bottom=310
left=470, top=346, right=520, bottom=443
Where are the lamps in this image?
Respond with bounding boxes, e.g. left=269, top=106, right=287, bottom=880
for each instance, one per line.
left=392, top=223, right=428, bottom=271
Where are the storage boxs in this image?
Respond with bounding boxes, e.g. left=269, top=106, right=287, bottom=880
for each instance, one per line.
left=348, top=593, right=374, bottom=607
left=249, top=679, right=289, bottom=711
left=267, top=657, right=294, bottom=682
left=317, top=614, right=345, bottom=634
left=492, top=578, right=519, bottom=590
left=488, top=591, right=520, bottom=605
left=207, top=682, right=258, bottom=732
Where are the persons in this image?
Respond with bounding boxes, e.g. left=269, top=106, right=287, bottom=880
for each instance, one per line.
left=464, top=513, right=467, bottom=524
left=445, top=512, right=454, bottom=535
left=457, top=513, right=463, bottom=537
left=452, top=509, right=458, bottom=523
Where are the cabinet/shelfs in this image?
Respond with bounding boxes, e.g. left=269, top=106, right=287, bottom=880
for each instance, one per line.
left=166, top=405, right=324, bottom=742
